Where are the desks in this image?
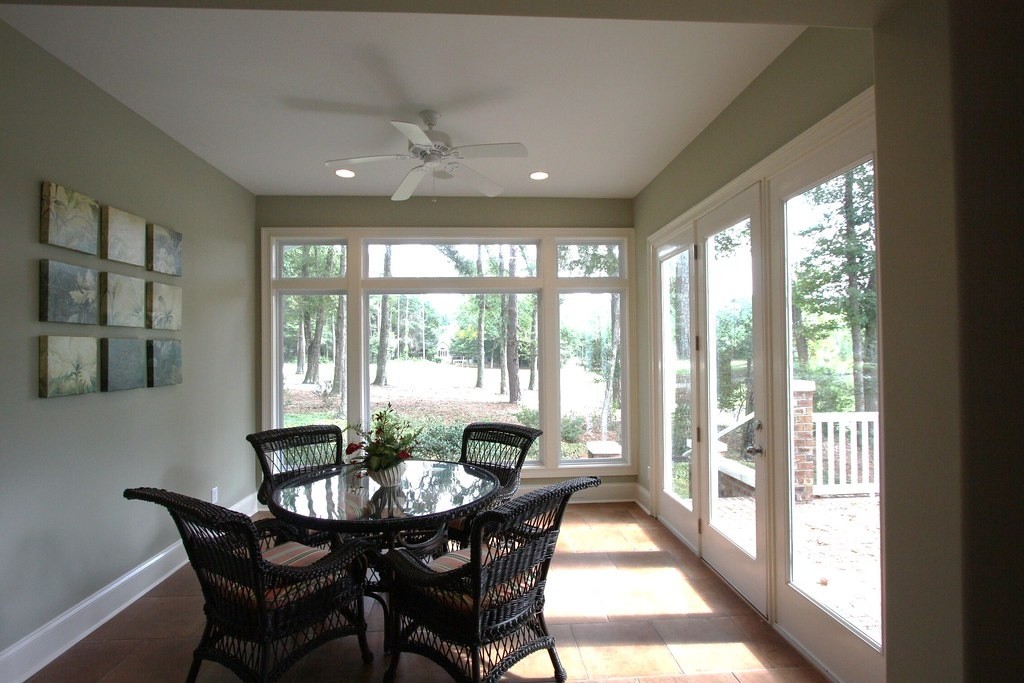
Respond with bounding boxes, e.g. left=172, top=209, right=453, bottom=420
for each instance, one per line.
left=266, top=457, right=501, bottom=652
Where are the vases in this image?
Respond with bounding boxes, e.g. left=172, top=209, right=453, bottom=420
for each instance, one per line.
left=365, top=460, right=410, bottom=488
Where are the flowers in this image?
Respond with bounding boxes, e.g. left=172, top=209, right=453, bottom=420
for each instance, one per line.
left=334, top=403, right=424, bottom=485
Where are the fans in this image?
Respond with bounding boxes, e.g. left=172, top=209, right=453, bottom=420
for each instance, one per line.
left=321, top=109, right=529, bottom=201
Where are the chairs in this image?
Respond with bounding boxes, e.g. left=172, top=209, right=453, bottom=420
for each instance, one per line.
left=122, top=487, right=375, bottom=683
left=426, top=420, right=544, bottom=558
left=246, top=423, right=357, bottom=550
left=376, top=475, right=603, bottom=683
